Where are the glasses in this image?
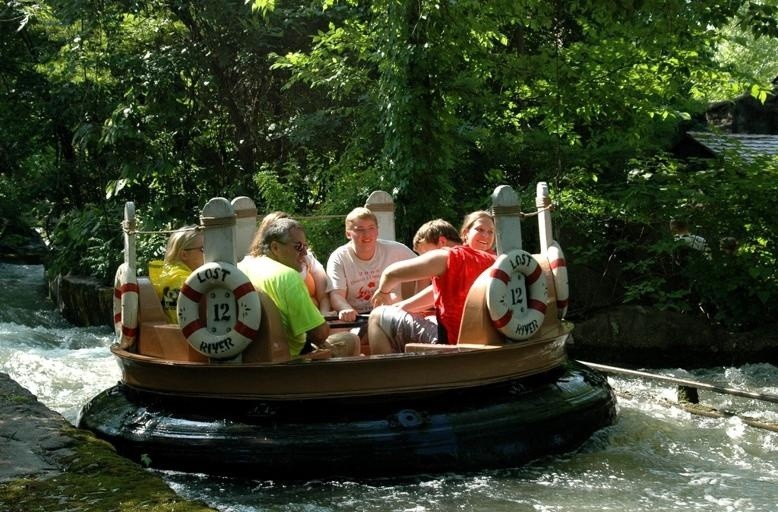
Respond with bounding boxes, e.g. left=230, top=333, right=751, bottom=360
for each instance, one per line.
left=287, top=241, right=309, bottom=253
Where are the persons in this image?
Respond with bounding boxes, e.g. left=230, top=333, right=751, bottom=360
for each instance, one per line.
left=668, top=215, right=738, bottom=259
left=236, top=206, right=497, bottom=358
left=149, top=226, right=204, bottom=325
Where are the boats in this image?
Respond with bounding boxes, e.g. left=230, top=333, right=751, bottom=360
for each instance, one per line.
left=77, top=182, right=617, bottom=487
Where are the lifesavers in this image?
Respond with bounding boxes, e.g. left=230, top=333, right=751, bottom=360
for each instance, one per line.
left=483, top=249, right=549, bottom=337
left=113, top=263, right=138, bottom=349
left=175, top=261, right=261, bottom=359
left=549, top=240, right=571, bottom=318
